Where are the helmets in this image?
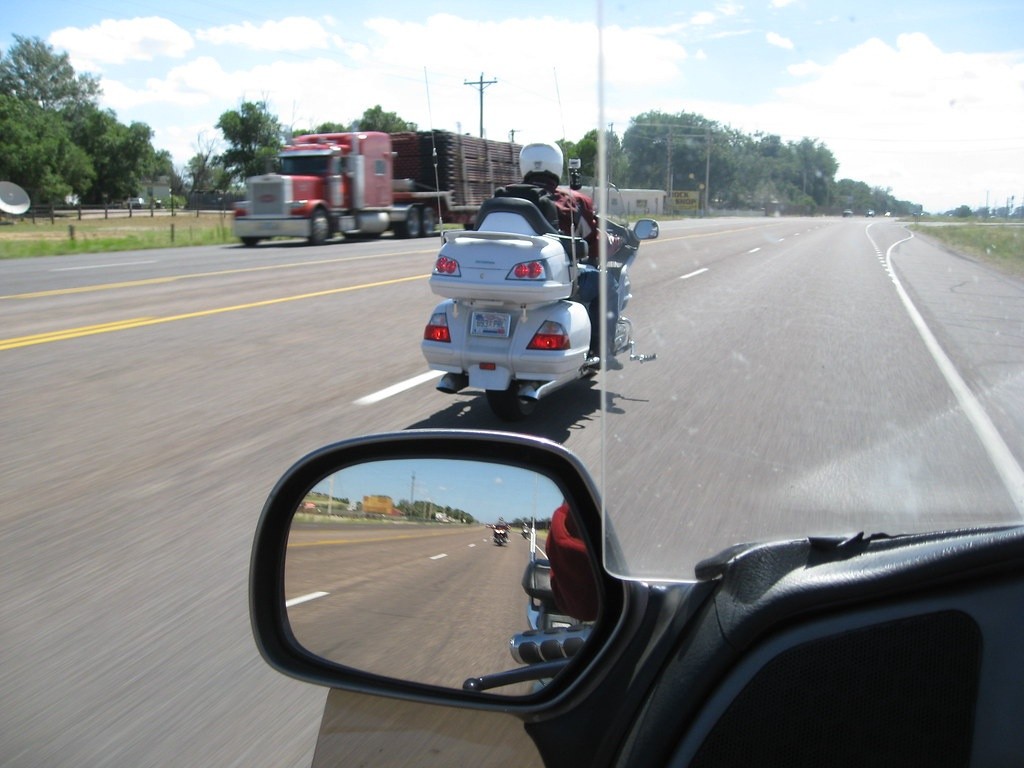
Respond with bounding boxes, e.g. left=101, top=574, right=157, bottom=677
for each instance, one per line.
left=519, top=139, right=564, bottom=185
left=499, top=517, right=503, bottom=521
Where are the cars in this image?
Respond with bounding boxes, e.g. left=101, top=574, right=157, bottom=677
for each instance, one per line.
left=885, top=212, right=893, bottom=216
left=843, top=209, right=855, bottom=217
left=866, top=210, right=876, bottom=217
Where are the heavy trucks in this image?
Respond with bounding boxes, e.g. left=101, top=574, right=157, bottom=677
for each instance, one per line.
left=231, top=129, right=523, bottom=247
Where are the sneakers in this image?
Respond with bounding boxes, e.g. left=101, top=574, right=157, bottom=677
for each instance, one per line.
left=590, top=353, right=623, bottom=371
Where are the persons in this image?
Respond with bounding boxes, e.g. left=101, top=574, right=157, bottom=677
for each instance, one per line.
left=522, top=523, right=529, bottom=530
left=493, top=517, right=510, bottom=540
left=519, top=140, right=626, bottom=370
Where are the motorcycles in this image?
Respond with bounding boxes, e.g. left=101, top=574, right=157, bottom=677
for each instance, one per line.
left=492, top=524, right=511, bottom=546
left=420, top=173, right=660, bottom=421
